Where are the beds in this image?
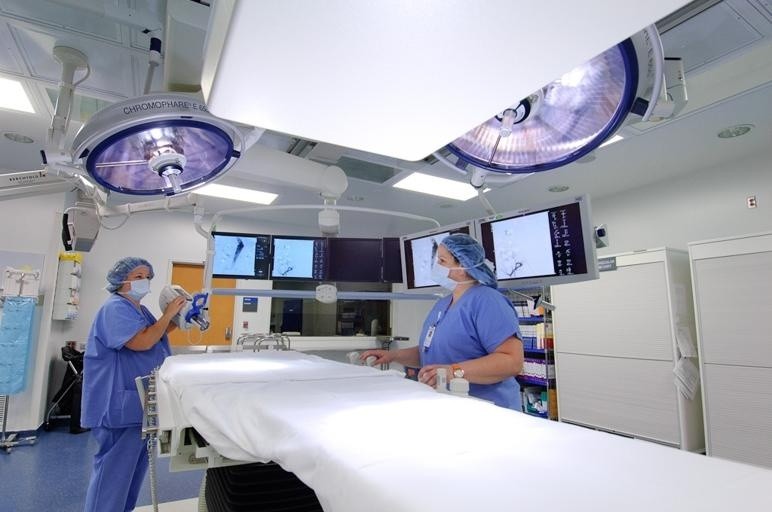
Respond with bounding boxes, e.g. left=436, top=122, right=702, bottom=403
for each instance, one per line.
left=132, top=347, right=719, bottom=512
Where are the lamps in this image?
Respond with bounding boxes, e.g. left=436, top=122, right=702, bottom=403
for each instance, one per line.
left=444, top=43, right=637, bottom=189
left=61, top=96, right=246, bottom=206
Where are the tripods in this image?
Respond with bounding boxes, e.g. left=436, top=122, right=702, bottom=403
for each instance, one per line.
left=399, top=219, right=475, bottom=295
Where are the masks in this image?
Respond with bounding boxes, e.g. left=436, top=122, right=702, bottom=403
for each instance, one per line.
left=116, top=278, right=151, bottom=300
left=430, top=262, right=459, bottom=290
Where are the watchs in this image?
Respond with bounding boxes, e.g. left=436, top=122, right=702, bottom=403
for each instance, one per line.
left=450, top=362, right=467, bottom=381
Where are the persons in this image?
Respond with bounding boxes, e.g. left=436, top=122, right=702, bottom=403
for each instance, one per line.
left=352, top=305, right=384, bottom=337
left=76, top=253, right=189, bottom=512
left=356, top=231, right=528, bottom=414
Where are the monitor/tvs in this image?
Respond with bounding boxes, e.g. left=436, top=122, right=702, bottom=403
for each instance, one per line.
left=475, top=192, right=600, bottom=289
left=269, top=235, right=327, bottom=281
left=211, top=231, right=270, bottom=280
left=327, top=238, right=382, bottom=281
